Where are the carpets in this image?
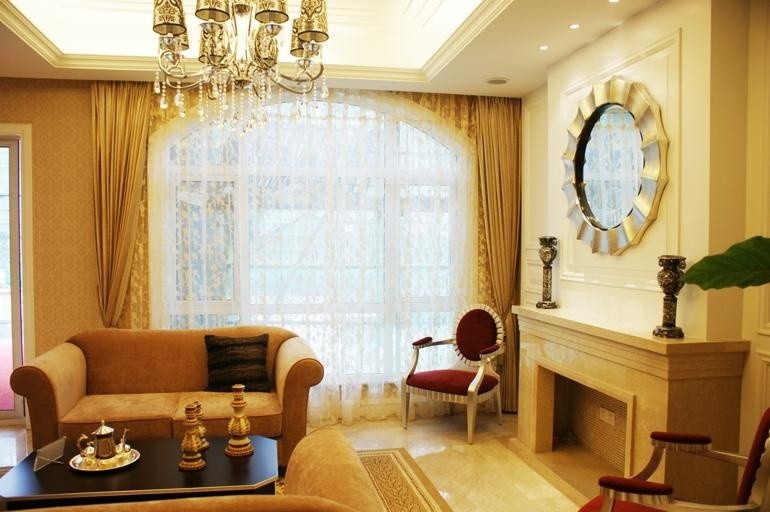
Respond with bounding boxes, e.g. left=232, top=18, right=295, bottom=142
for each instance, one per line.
left=358, top=446, right=453, bottom=511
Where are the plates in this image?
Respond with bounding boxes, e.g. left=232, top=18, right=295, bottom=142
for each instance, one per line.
left=68, top=448, right=141, bottom=471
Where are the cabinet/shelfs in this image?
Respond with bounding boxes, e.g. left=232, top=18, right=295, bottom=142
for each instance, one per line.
left=511, top=300, right=751, bottom=505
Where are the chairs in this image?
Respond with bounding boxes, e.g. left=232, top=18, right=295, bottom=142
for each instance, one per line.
left=402, top=303, right=507, bottom=445
left=578, top=407, right=770, bottom=512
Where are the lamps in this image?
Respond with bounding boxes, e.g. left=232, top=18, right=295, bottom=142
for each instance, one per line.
left=150, top=0, right=329, bottom=132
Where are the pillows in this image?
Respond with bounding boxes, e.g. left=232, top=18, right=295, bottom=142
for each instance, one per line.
left=204, top=333, right=271, bottom=391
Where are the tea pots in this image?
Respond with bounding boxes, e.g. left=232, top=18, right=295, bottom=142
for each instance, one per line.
left=76, top=419, right=130, bottom=459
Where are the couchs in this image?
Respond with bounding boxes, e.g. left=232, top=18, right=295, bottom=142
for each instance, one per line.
left=11, top=327, right=324, bottom=466
left=19, top=427, right=387, bottom=512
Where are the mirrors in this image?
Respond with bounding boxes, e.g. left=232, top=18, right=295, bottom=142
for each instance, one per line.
left=561, top=74, right=669, bottom=255
